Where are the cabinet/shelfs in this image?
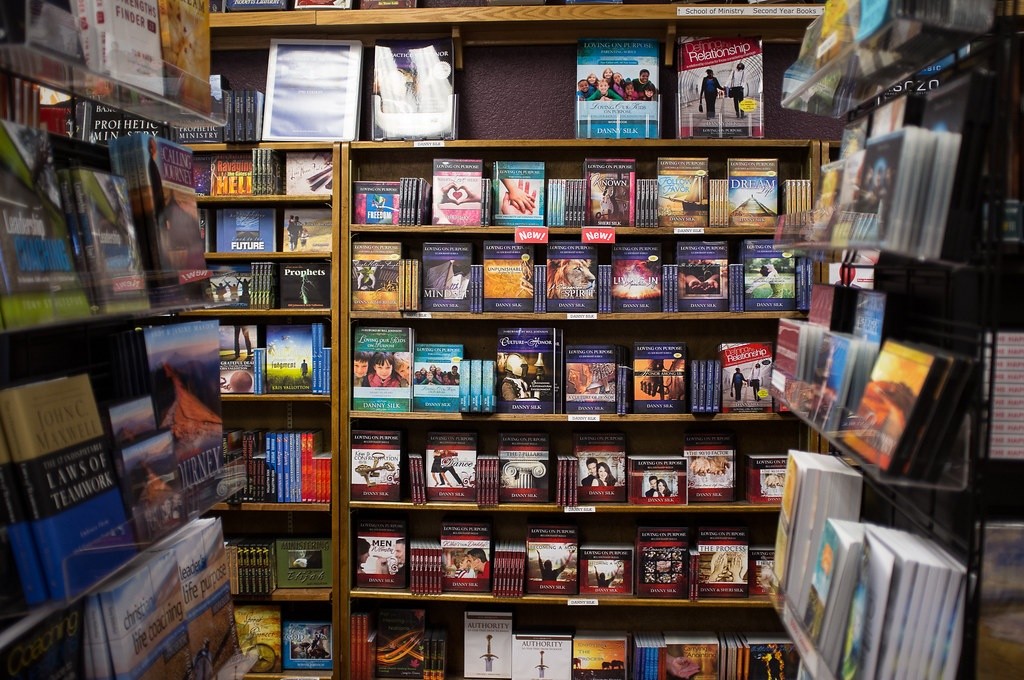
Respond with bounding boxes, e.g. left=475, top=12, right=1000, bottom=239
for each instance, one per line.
left=0, top=3, right=1024, bottom=680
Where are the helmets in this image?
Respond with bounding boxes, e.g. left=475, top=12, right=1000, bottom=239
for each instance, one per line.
left=505, top=353, right=529, bottom=375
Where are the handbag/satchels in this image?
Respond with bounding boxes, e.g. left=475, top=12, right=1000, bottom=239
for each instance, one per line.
left=728, top=89, right=734, bottom=98
left=699, top=99, right=703, bottom=112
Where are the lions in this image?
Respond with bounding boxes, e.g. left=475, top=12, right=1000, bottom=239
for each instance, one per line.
left=547, top=258, right=596, bottom=300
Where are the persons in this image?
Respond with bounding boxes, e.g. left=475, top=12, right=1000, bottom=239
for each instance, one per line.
left=501, top=179, right=535, bottom=213
left=354, top=351, right=409, bottom=387
left=582, top=457, right=625, bottom=486
left=414, top=365, right=460, bottom=385
left=700, top=63, right=745, bottom=118
left=358, top=538, right=405, bottom=574
left=731, top=364, right=761, bottom=400
left=577, top=69, right=655, bottom=101
left=454, top=548, right=489, bottom=578
left=502, top=180, right=536, bottom=216
left=645, top=476, right=675, bottom=498
left=431, top=450, right=464, bottom=487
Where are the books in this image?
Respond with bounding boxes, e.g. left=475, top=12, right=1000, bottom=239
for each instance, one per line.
left=722, top=341, right=773, bottom=413
left=408, top=536, right=442, bottom=595
left=478, top=433, right=579, bottom=507
left=355, top=520, right=408, bottom=590
left=352, top=240, right=811, bottom=311
left=684, top=434, right=789, bottom=504
left=352, top=327, right=413, bottom=412
left=577, top=432, right=626, bottom=502
left=353, top=157, right=812, bottom=228
left=224, top=1, right=461, bottom=142
left=576, top=38, right=661, bottom=138
left=441, top=522, right=491, bottom=593
left=631, top=458, right=689, bottom=505
left=494, top=527, right=775, bottom=598
left=351, top=430, right=427, bottom=504
left=461, top=328, right=720, bottom=413
left=351, top=608, right=799, bottom=680
left=769, top=0, right=1024, bottom=680
left=676, top=36, right=764, bottom=138
left=427, top=427, right=477, bottom=501
left=414, top=344, right=463, bottom=412
left=0, top=0, right=331, bottom=680
